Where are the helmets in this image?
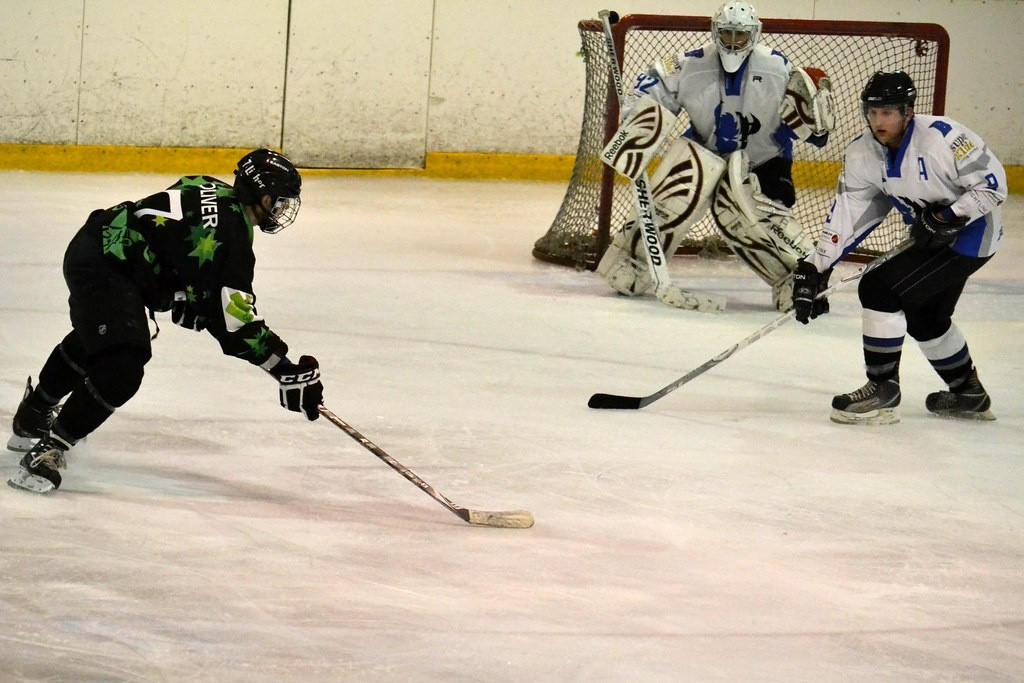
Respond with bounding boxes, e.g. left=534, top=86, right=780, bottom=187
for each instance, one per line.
left=861, top=71, right=917, bottom=108
left=233, top=148, right=302, bottom=234
left=711, top=1, right=763, bottom=73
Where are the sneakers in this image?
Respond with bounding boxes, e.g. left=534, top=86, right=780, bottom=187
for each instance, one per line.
left=830, top=361, right=902, bottom=425
left=7, top=427, right=72, bottom=497
left=7, top=375, right=65, bottom=454
left=925, top=365, right=997, bottom=420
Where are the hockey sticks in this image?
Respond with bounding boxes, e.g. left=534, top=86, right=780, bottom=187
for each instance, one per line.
left=596, top=8, right=731, bottom=313
left=585, top=240, right=913, bottom=410
left=313, top=401, right=534, bottom=531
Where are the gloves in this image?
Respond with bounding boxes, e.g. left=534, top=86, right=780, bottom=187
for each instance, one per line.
left=910, top=201, right=971, bottom=253
left=270, top=354, right=324, bottom=421
left=172, top=300, right=207, bottom=332
left=791, top=254, right=833, bottom=325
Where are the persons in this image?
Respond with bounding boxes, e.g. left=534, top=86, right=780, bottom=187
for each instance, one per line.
left=593, top=0, right=835, bottom=297
left=5, top=146, right=324, bottom=496
left=791, top=68, right=1009, bottom=425
